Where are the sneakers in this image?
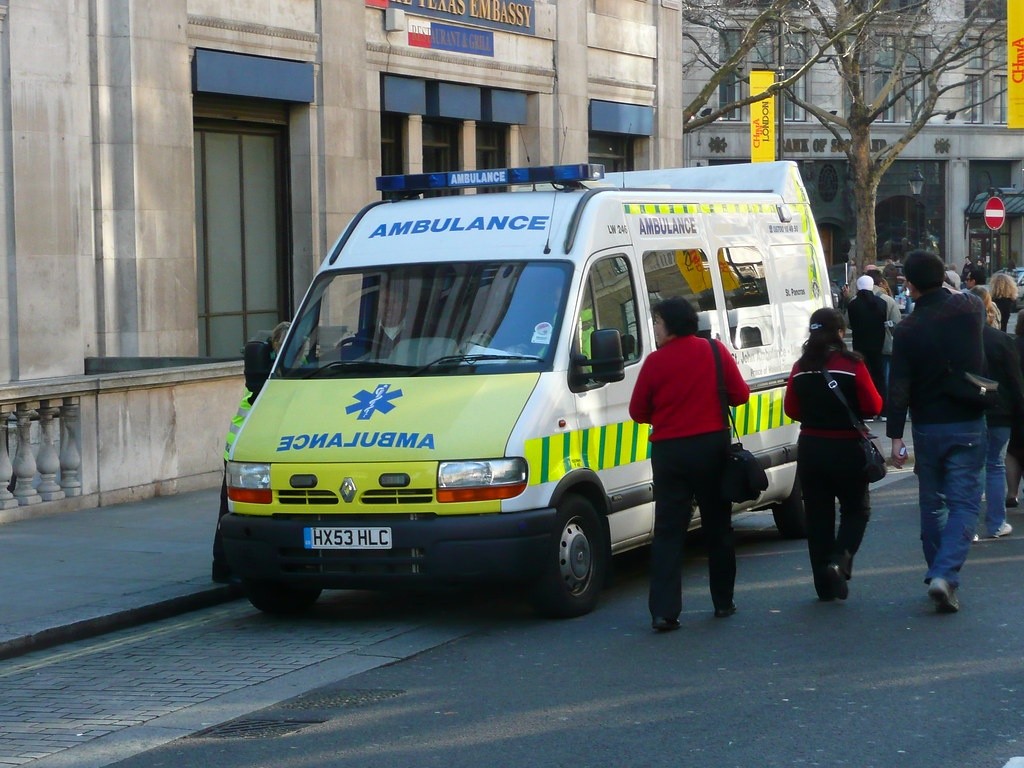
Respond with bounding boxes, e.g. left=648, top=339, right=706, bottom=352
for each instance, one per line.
left=928, top=578, right=960, bottom=614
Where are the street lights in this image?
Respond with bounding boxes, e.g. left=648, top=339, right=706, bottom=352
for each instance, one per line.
left=907, top=166, right=925, bottom=248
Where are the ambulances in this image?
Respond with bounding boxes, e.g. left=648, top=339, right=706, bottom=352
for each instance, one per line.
left=220, top=161, right=837, bottom=615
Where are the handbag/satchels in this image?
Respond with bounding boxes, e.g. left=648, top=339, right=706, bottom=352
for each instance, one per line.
left=722, top=443, right=770, bottom=503
left=858, top=433, right=888, bottom=483
left=882, top=327, right=894, bottom=356
left=942, top=371, right=1000, bottom=410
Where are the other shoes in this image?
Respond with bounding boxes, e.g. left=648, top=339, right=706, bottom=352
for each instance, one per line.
left=715, top=601, right=738, bottom=615
left=653, top=616, right=682, bottom=631
left=1006, top=495, right=1019, bottom=506
left=815, top=564, right=850, bottom=602
left=992, top=523, right=1013, bottom=538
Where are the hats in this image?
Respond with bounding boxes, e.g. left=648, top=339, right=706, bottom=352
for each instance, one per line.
left=857, top=274, right=876, bottom=291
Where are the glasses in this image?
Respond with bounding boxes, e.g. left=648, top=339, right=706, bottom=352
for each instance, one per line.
left=966, top=278, right=972, bottom=282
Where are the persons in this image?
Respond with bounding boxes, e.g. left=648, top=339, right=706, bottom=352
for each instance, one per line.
left=784, top=307, right=883, bottom=601
left=211, top=316, right=321, bottom=583
left=887, top=251, right=989, bottom=615
left=832, top=256, right=1024, bottom=544
left=349, top=279, right=429, bottom=360
left=628, top=295, right=750, bottom=632
left=504, top=277, right=596, bottom=374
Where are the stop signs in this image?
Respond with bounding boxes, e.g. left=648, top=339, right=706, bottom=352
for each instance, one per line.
left=982, top=196, right=1006, bottom=230
